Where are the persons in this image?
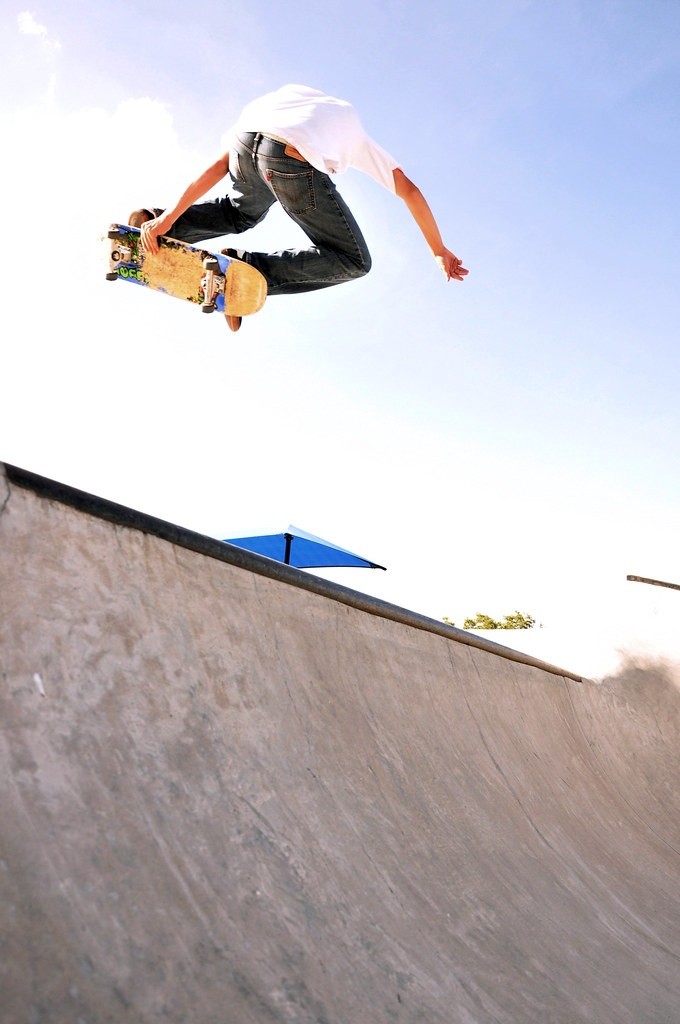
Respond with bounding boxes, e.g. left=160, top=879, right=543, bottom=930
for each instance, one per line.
left=130, top=84, right=468, bottom=332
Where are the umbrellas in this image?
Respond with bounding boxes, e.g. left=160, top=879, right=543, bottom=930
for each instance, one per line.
left=221, top=523, right=385, bottom=570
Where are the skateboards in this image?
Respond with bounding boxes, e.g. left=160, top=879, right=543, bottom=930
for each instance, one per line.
left=99, top=224, right=268, bottom=317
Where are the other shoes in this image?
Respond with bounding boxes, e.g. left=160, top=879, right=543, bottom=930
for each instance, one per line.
left=128, top=209, right=157, bottom=230
left=220, top=248, right=247, bottom=332
